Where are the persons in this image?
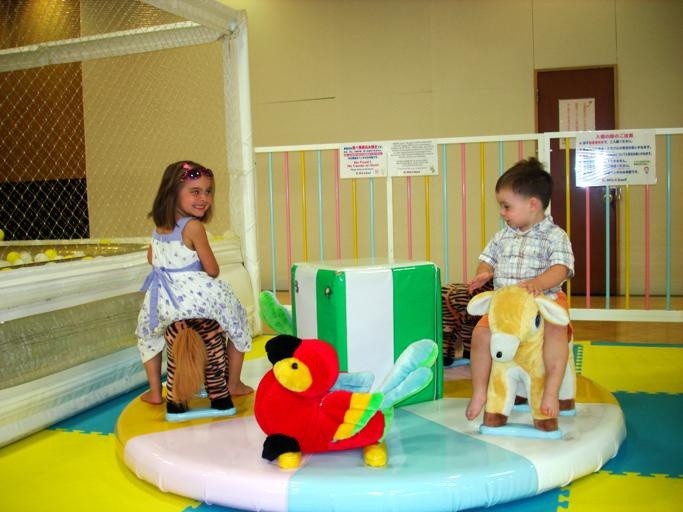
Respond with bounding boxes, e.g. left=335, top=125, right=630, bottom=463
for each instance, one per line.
left=467, top=162, right=574, bottom=421
left=134, top=161, right=254, bottom=406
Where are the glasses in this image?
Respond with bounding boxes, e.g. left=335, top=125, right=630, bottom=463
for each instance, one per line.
left=181, top=168, right=214, bottom=180
